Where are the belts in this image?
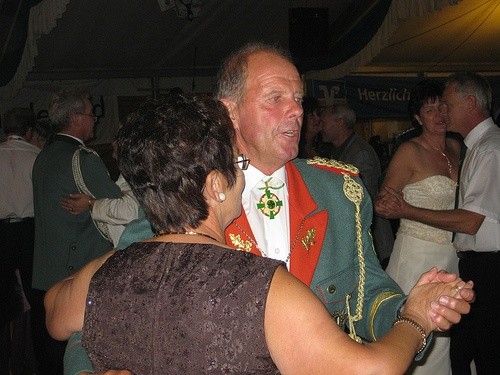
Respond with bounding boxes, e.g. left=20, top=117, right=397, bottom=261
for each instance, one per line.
left=456, top=251, right=500, bottom=260
left=0, top=218, right=34, bottom=224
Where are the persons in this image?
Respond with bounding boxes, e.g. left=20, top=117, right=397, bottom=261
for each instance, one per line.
left=317, top=104, right=395, bottom=271
left=33, top=90, right=124, bottom=375
left=59, top=121, right=140, bottom=250
left=374, top=71, right=500, bottom=375
left=45, top=87, right=473, bottom=375
left=0, top=107, right=56, bottom=308
left=64, top=40, right=476, bottom=375
left=299, top=96, right=320, bottom=158
left=378, top=84, right=476, bottom=375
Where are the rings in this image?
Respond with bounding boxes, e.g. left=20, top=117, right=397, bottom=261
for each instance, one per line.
left=454, top=285, right=461, bottom=292
left=71, top=208, right=74, bottom=212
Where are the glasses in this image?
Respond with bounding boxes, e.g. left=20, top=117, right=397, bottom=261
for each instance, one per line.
left=233, top=154, right=250, bottom=170
left=306, top=115, right=320, bottom=120
left=76, top=112, right=97, bottom=123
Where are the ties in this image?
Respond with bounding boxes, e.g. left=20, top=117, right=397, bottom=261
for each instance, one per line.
left=452, top=142, right=466, bottom=244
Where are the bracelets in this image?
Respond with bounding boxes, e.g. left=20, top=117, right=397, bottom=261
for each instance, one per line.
left=392, top=318, right=427, bottom=353
left=88, top=197, right=92, bottom=209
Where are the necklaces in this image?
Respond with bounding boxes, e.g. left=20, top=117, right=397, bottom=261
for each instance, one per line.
left=153, top=232, right=220, bottom=243
left=435, top=145, right=452, bottom=174
